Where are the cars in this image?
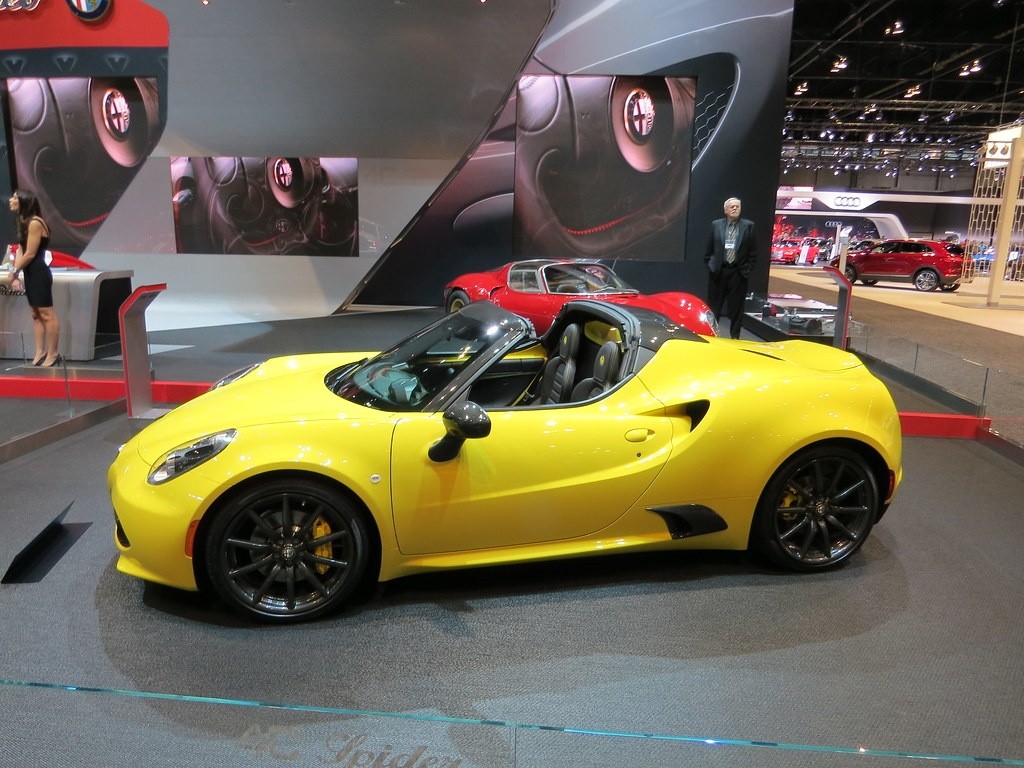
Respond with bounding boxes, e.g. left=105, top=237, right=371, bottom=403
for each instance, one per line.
left=829, top=238, right=975, bottom=292
left=770, top=237, right=830, bottom=264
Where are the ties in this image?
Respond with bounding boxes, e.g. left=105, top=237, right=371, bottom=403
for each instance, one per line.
left=728, top=224, right=735, bottom=263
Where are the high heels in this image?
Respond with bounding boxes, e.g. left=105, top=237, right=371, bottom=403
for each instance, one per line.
left=40, top=354, right=62, bottom=367
left=31, top=351, right=47, bottom=367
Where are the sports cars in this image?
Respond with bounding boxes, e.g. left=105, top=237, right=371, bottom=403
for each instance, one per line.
left=105, top=258, right=906, bottom=622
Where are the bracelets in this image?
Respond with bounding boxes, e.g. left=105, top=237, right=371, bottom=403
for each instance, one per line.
left=13, top=268, right=20, bottom=275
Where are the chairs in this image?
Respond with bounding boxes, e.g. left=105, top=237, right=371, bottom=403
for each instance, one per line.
left=527, top=299, right=639, bottom=407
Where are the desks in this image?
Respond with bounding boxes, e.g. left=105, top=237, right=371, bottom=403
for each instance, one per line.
left=0, top=268, right=133, bottom=361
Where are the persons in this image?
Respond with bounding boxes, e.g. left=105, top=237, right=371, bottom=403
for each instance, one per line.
left=7, top=189, right=62, bottom=367
left=703, top=198, right=757, bottom=341
left=963, top=239, right=984, bottom=253
